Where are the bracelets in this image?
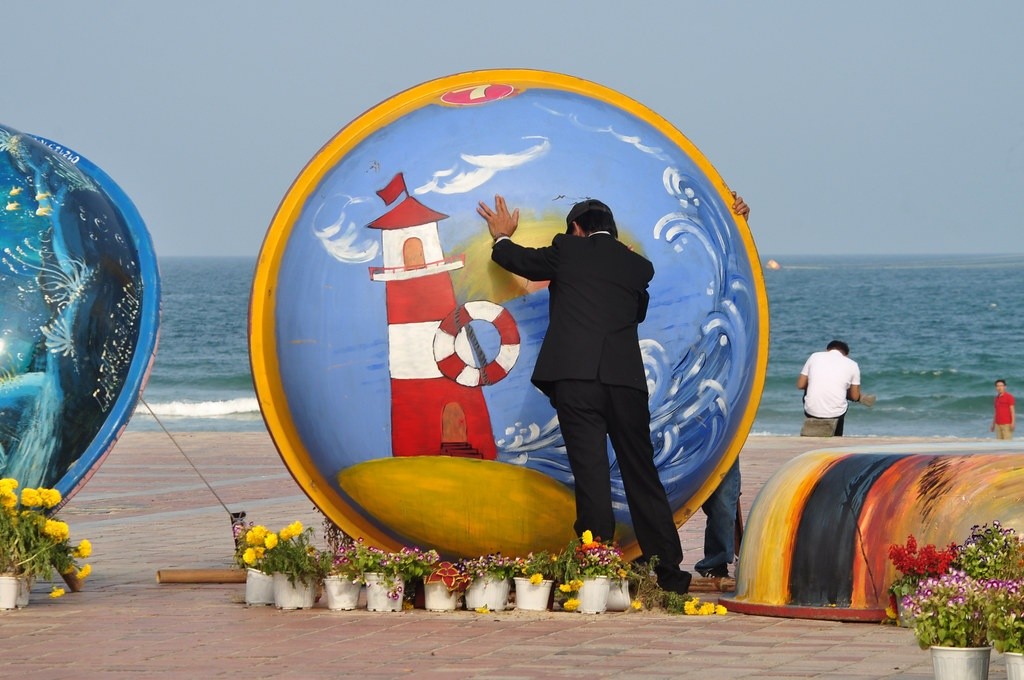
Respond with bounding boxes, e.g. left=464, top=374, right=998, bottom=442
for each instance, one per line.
left=493, top=233, right=508, bottom=240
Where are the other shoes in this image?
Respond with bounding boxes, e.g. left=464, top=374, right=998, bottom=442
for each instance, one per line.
left=707, top=568, right=732, bottom=579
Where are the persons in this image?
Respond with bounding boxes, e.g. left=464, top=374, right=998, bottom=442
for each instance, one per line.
left=477, top=193, right=691, bottom=596
left=798, top=341, right=875, bottom=436
left=695, top=192, right=749, bottom=579
left=991, top=380, right=1015, bottom=439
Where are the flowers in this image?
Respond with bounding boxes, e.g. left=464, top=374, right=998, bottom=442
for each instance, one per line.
left=0, top=478, right=92, bottom=599
left=234, top=506, right=730, bottom=615
left=884, top=520, right=1024, bottom=655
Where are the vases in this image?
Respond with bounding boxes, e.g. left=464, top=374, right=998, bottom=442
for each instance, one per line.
left=931, top=646, right=991, bottom=680
left=414, top=581, right=426, bottom=609
left=895, top=591, right=915, bottom=628
left=244, top=568, right=274, bottom=606
left=15, top=579, right=29, bottom=610
left=363, top=572, right=405, bottom=613
left=1003, top=652, right=1024, bottom=680
left=577, top=574, right=612, bottom=615
left=0, top=577, right=21, bottom=611
left=607, top=576, right=631, bottom=612
left=271, top=570, right=317, bottom=611
left=513, top=577, right=553, bottom=612
left=323, top=575, right=361, bottom=610
left=423, top=575, right=456, bottom=613
left=464, top=576, right=510, bottom=611
